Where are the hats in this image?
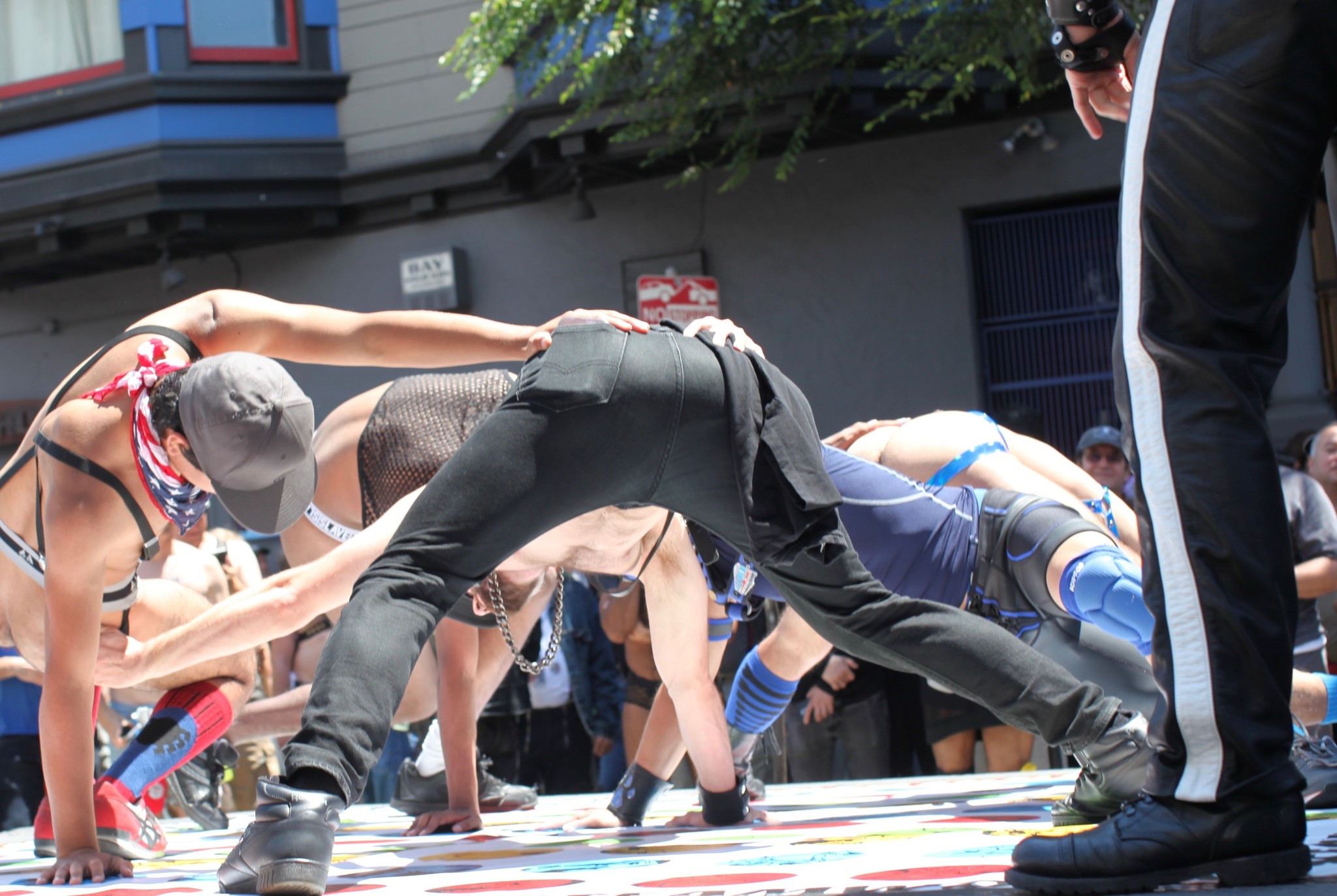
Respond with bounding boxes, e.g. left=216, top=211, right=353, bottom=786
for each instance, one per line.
left=1075, top=427, right=1123, bottom=456
left=178, top=350, right=319, bottom=533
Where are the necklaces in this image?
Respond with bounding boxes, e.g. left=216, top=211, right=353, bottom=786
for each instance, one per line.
left=489, top=567, right=566, bottom=675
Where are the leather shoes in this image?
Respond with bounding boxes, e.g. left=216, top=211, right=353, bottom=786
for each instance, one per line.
left=167, top=738, right=238, bottom=830
left=1003, top=790, right=1312, bottom=892
left=389, top=750, right=537, bottom=812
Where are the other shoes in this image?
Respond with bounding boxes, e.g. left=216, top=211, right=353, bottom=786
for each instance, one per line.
left=695, top=761, right=767, bottom=804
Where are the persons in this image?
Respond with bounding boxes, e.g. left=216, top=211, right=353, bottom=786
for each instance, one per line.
left=0, top=288, right=1337, bottom=896
left=1005, top=0, right=1337, bottom=896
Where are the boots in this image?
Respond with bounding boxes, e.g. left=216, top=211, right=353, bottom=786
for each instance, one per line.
left=1049, top=711, right=1180, bottom=827
left=218, top=776, right=340, bottom=894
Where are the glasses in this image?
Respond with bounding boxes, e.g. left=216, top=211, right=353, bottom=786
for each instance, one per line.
left=1083, top=452, right=1125, bottom=463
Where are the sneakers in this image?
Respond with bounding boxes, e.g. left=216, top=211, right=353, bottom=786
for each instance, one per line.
left=1289, top=713, right=1337, bottom=809
left=33, top=781, right=58, bottom=858
left=93, top=779, right=166, bottom=857
